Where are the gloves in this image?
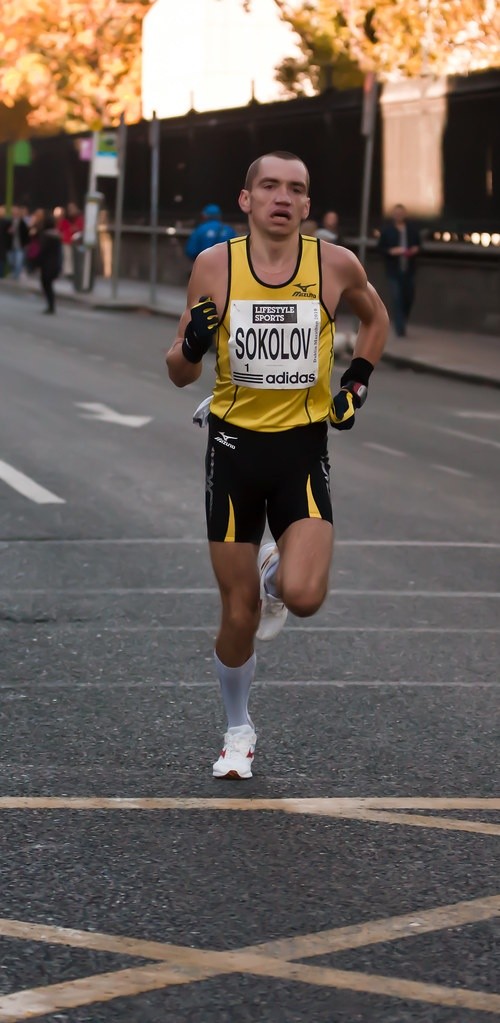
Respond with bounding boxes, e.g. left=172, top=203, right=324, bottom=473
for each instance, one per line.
left=328, top=389, right=356, bottom=431
left=182, top=294, right=220, bottom=365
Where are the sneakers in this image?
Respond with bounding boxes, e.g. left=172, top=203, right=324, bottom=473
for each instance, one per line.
left=211, top=724, right=257, bottom=780
left=254, top=543, right=289, bottom=642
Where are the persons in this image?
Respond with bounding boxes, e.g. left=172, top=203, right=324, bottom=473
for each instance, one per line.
left=184, top=204, right=237, bottom=259
left=0, top=201, right=84, bottom=314
left=378, top=204, right=420, bottom=338
left=165, top=151, right=390, bottom=780
left=300, top=211, right=340, bottom=243
